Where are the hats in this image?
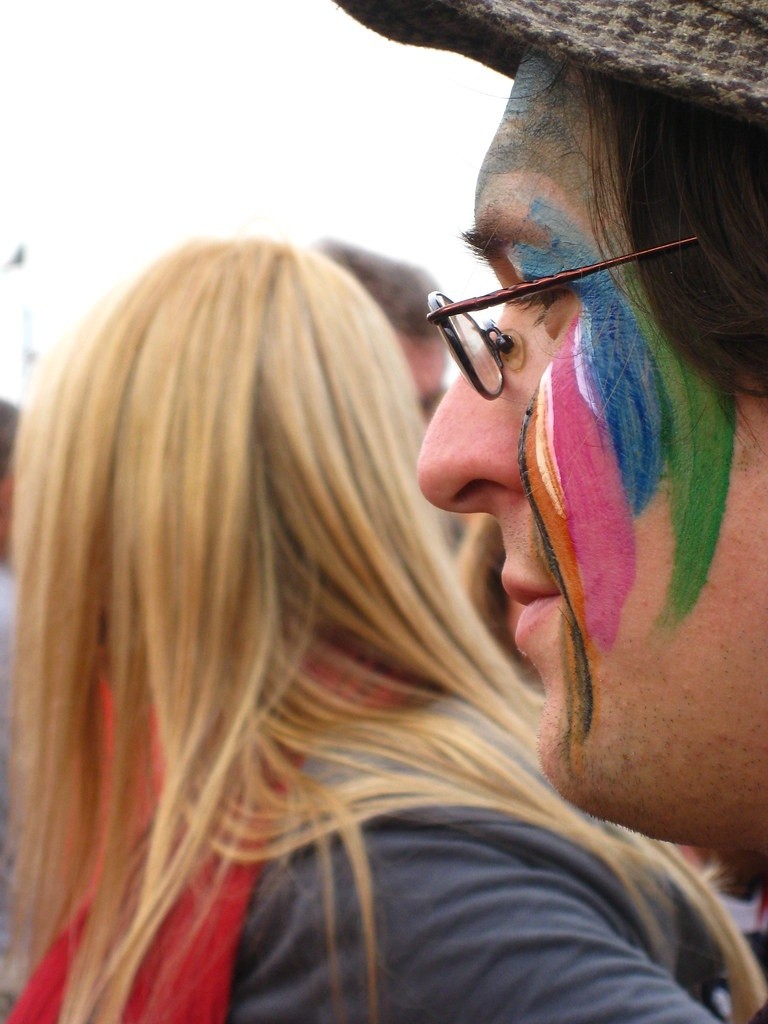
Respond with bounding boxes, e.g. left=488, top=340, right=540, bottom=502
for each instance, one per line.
left=334, top=0, right=768, bottom=125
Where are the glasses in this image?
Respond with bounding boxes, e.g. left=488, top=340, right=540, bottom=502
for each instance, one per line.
left=427, top=237, right=698, bottom=401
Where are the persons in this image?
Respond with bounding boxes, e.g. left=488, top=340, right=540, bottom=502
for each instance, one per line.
left=1, top=0, right=768, bottom=1023
